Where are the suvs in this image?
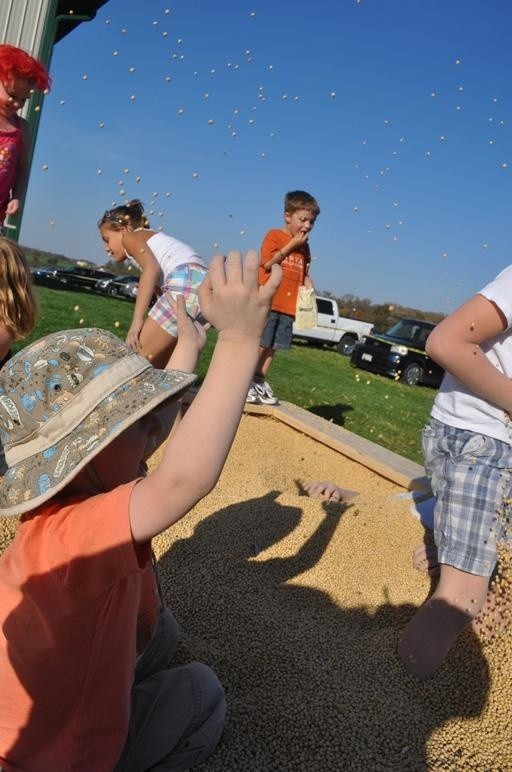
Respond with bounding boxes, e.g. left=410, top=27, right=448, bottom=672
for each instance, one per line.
left=351, top=318, right=449, bottom=388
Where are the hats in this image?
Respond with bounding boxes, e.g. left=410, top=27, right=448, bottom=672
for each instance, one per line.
left=0, top=327, right=197, bottom=516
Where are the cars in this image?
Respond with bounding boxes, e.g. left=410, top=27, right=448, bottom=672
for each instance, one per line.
left=29, top=262, right=157, bottom=307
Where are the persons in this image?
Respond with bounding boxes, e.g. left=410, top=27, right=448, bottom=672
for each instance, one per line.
left=0, top=44, right=52, bottom=226
left=245, top=191, right=320, bottom=406
left=98, top=199, right=210, bottom=369
left=0, top=237, right=38, bottom=362
left=0, top=249, right=283, bottom=771
left=300, top=480, right=440, bottom=579
left=397, top=265, right=512, bottom=679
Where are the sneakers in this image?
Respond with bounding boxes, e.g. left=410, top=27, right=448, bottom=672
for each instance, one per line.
left=246, top=380, right=278, bottom=405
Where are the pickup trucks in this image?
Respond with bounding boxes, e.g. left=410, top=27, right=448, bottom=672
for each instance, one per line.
left=292, top=294, right=374, bottom=355
